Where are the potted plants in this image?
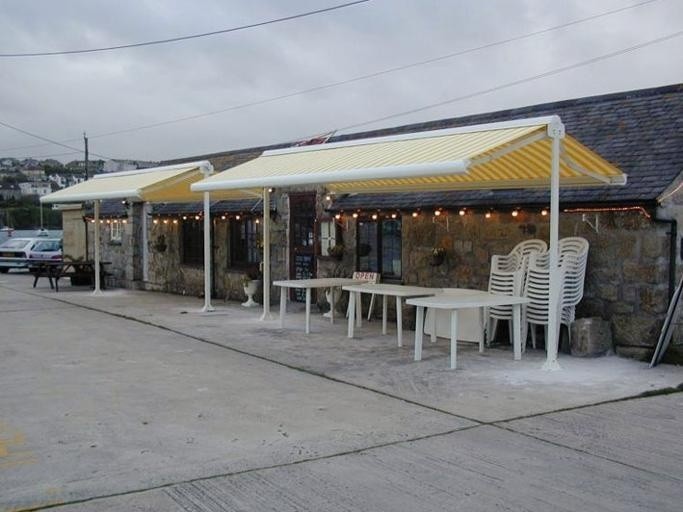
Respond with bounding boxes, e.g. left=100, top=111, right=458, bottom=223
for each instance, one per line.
left=239, top=268, right=262, bottom=307
left=321, top=267, right=352, bottom=317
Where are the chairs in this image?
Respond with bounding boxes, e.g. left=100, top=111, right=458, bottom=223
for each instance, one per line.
left=484, top=236, right=591, bottom=353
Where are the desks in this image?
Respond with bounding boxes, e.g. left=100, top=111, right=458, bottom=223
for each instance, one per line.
left=32, top=261, right=112, bottom=289
left=271, top=278, right=529, bottom=373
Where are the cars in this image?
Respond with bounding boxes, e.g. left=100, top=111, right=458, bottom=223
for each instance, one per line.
left=0, top=238, right=63, bottom=273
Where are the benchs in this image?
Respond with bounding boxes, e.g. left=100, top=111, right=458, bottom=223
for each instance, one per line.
left=31, top=272, right=113, bottom=292
